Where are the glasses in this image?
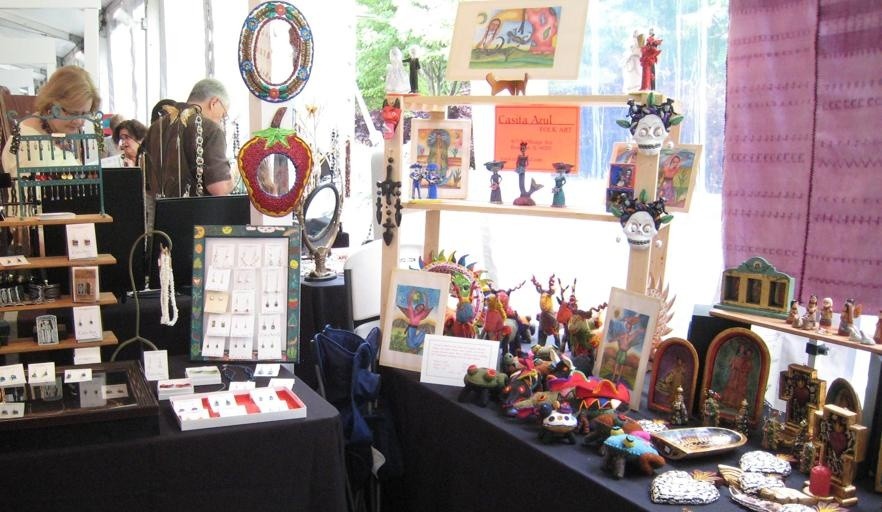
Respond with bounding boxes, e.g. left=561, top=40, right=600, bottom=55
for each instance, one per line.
left=220, top=101, right=229, bottom=122
left=61, top=107, right=91, bottom=117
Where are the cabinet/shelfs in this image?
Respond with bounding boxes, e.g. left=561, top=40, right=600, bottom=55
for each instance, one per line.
left=379, top=92, right=683, bottom=357
left=0, top=213, right=119, bottom=354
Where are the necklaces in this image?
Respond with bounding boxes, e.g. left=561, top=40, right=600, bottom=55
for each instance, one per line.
left=157, top=250, right=178, bottom=326
left=36, top=114, right=77, bottom=152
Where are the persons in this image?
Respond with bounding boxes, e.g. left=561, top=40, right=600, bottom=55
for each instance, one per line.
left=1, top=64, right=101, bottom=178
left=100, top=94, right=180, bottom=167
left=135, top=76, right=241, bottom=199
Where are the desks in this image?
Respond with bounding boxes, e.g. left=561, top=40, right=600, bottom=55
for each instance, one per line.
left=1, top=359, right=351, bottom=510
left=300, top=275, right=347, bottom=329
left=0, top=288, right=191, bottom=365
left=373, top=346, right=880, bottom=511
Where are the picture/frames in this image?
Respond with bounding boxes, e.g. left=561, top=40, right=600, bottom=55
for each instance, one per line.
left=190, top=223, right=304, bottom=365
left=700, top=327, right=771, bottom=428
left=595, top=287, right=661, bottom=410
left=379, top=268, right=451, bottom=370
left=647, top=338, right=699, bottom=418
